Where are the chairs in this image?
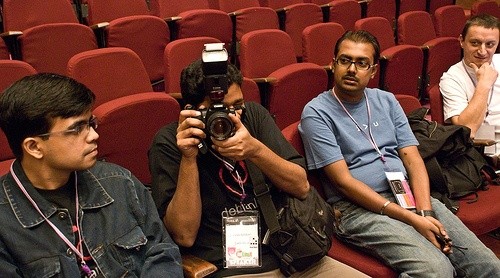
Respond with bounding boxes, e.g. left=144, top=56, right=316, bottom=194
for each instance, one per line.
left=1, top=0, right=500, bottom=278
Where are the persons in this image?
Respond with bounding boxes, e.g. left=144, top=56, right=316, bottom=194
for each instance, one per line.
left=0, top=73, right=183, bottom=278
left=439, top=14, right=500, bottom=182
left=148, top=59, right=371, bottom=278
left=299, top=30, right=500, bottom=278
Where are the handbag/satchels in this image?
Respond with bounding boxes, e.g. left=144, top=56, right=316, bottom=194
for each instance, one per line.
left=262, top=185, right=336, bottom=278
left=404, top=105, right=499, bottom=214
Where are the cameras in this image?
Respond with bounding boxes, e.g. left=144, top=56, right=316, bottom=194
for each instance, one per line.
left=188, top=42, right=246, bottom=141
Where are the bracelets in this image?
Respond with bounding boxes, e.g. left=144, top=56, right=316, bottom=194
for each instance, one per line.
left=416, top=209, right=436, bottom=218
left=380, top=200, right=392, bottom=216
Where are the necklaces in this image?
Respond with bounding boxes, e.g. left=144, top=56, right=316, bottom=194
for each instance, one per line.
left=9, top=160, right=96, bottom=278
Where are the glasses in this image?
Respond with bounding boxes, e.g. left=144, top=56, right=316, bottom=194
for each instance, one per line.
left=37, top=111, right=97, bottom=141
left=333, top=56, right=377, bottom=72
